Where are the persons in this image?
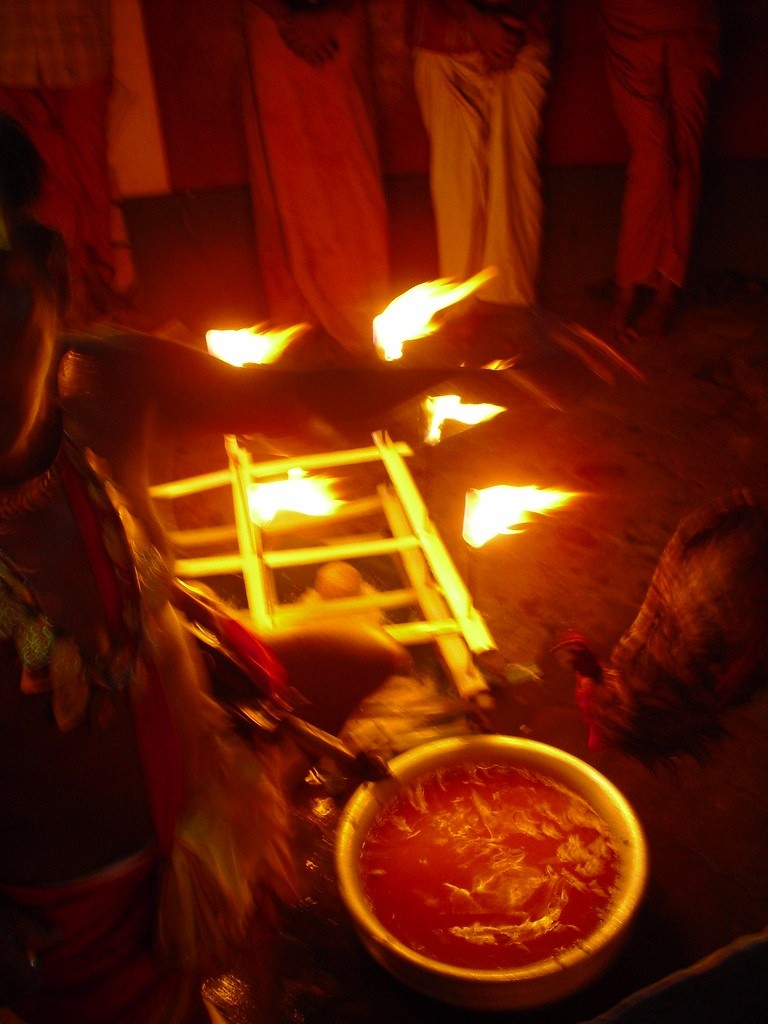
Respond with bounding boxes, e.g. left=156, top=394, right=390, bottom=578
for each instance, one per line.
left=224, top=0, right=395, bottom=366
left=407, top=1, right=554, bottom=346
left=3, top=0, right=139, bottom=301
left=1, top=133, right=661, bottom=1023
left=603, top=0, right=718, bottom=336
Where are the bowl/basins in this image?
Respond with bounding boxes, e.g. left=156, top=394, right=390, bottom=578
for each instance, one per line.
left=336, top=734, right=649, bottom=1012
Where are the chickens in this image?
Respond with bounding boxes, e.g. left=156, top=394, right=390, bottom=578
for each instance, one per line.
left=545, top=479, right=768, bottom=780
